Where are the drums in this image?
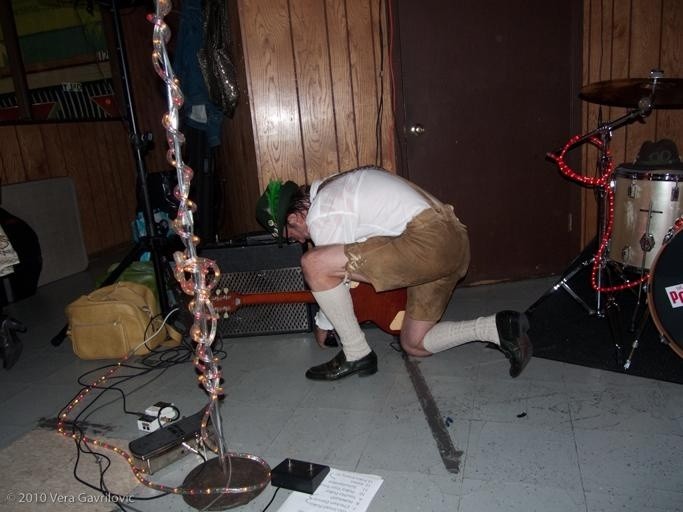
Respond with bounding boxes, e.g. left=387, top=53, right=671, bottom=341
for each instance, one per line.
left=603, top=163, right=683, bottom=275
left=643, top=215, right=683, bottom=359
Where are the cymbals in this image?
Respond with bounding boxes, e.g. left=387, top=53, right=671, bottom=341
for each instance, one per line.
left=579, top=78, right=682, bottom=109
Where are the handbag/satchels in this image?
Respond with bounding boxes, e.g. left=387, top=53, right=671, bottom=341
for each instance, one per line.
left=64, top=281, right=182, bottom=360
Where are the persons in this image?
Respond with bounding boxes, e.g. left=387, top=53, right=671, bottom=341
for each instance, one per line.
left=255, top=165, right=534, bottom=381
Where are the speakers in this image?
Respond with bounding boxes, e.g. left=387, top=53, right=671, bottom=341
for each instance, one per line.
left=188, top=241, right=314, bottom=338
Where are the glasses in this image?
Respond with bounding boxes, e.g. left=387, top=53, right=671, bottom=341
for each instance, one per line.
left=284, top=225, right=297, bottom=246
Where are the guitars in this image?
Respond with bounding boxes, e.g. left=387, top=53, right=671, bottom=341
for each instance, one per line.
left=188, top=280, right=407, bottom=335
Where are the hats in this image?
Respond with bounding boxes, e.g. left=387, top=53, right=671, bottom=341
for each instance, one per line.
left=255, top=180, right=298, bottom=249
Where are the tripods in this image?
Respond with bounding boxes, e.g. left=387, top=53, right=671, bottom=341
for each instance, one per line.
left=534, top=121, right=644, bottom=320
left=51, top=3, right=186, bottom=346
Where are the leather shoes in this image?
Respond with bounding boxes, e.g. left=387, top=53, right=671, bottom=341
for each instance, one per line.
left=495, top=310, right=533, bottom=379
left=305, top=349, right=378, bottom=381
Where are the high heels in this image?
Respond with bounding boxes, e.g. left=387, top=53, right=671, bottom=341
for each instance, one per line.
left=0, top=317, right=28, bottom=370
